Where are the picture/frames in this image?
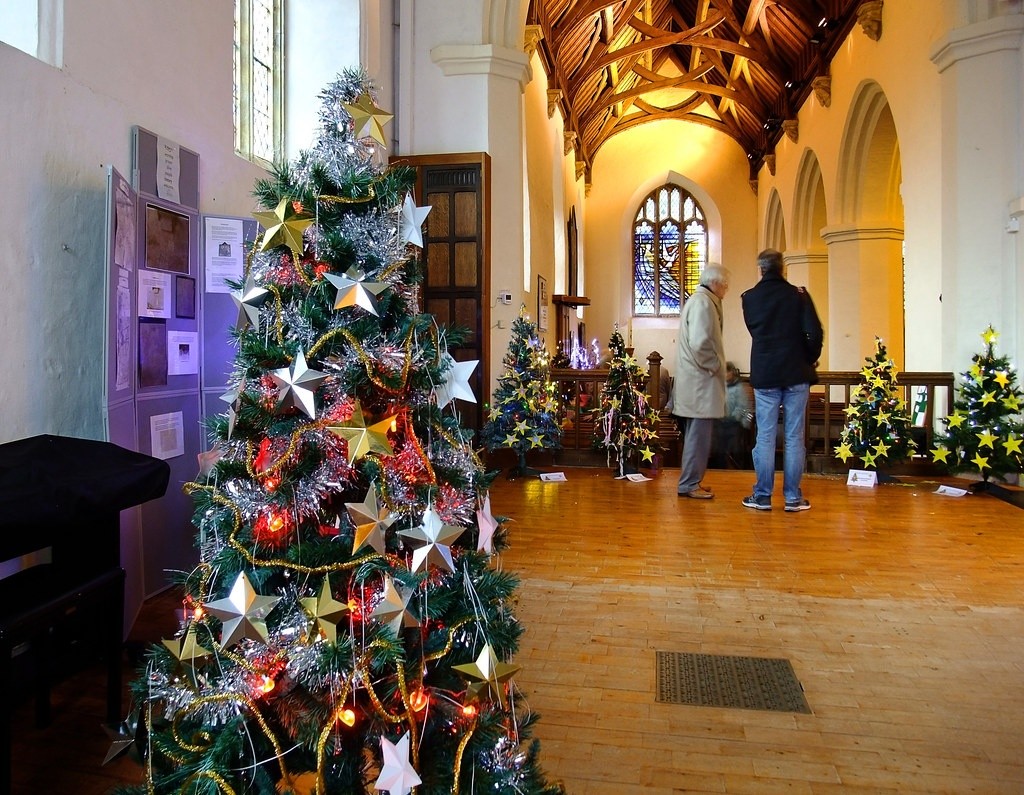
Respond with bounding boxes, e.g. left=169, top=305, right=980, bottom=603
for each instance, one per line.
left=580, top=322, right=587, bottom=349
left=568, top=205, right=578, bottom=309
left=538, top=275, right=549, bottom=333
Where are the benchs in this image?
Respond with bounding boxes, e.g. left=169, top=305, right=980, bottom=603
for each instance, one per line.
left=557, top=392, right=860, bottom=466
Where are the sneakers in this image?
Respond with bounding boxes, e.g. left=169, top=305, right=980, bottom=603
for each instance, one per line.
left=742, top=493, right=772, bottom=510
left=784, top=499, right=812, bottom=511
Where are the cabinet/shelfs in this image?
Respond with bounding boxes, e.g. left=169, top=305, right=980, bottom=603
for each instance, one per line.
left=0, top=434, right=170, bottom=795
left=389, top=152, right=491, bottom=467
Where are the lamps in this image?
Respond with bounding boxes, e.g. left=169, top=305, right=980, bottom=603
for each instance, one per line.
left=785, top=79, right=810, bottom=88
left=763, top=115, right=784, bottom=132
left=748, top=148, right=764, bottom=160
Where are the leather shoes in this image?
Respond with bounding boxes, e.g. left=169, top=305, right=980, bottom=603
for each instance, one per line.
left=678, top=486, right=714, bottom=499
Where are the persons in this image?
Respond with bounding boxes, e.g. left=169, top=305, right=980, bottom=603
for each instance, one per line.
left=740, top=249, right=824, bottom=511
left=713, top=361, right=752, bottom=470
left=671, top=263, right=731, bottom=500
left=645, top=360, right=670, bottom=410
